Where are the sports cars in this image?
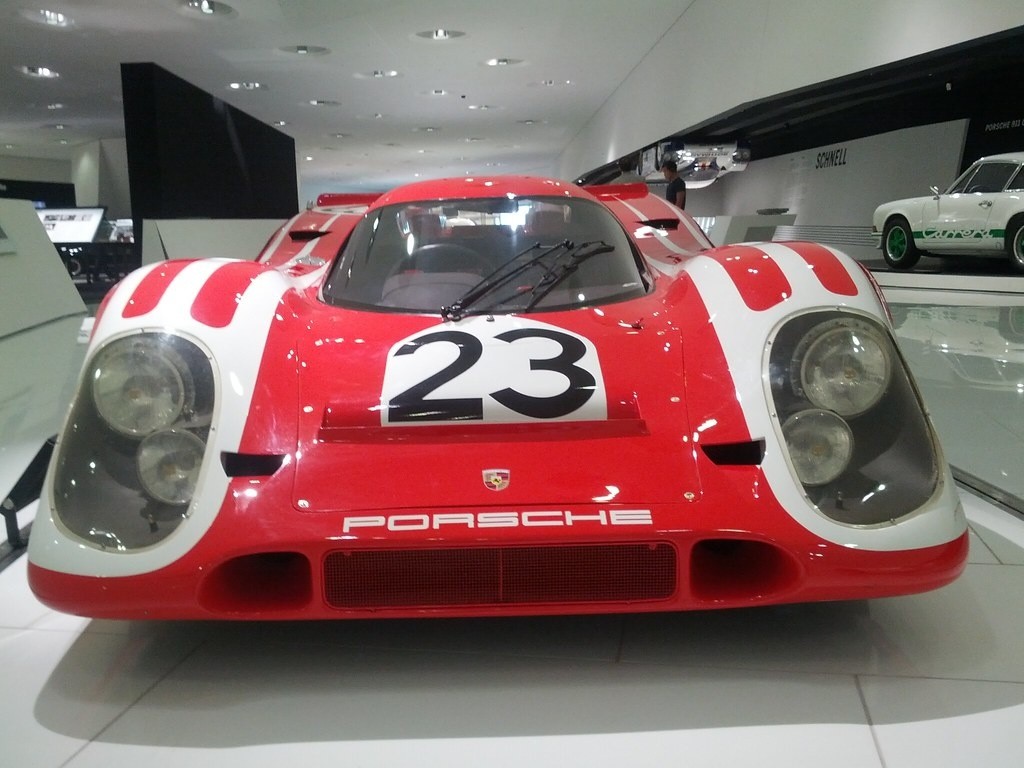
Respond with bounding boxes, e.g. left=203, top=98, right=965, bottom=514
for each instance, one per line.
left=27, top=175, right=970, bottom=624
left=636, top=137, right=751, bottom=189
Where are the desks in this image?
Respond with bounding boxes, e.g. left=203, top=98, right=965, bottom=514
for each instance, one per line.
left=52, top=242, right=135, bottom=284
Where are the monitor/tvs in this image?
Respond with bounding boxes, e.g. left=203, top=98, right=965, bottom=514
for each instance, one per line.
left=35, top=206, right=108, bottom=242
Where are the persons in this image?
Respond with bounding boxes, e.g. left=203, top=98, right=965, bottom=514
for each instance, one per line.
left=662, top=160, right=687, bottom=210
left=732, top=149, right=750, bottom=162
left=608, top=156, right=646, bottom=186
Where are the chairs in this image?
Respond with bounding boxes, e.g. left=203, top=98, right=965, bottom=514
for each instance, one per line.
left=415, top=233, right=513, bottom=276
left=86, top=253, right=120, bottom=286
left=522, top=234, right=619, bottom=292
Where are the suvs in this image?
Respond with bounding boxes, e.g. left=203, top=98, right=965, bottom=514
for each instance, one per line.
left=871, top=152, right=1024, bottom=271
